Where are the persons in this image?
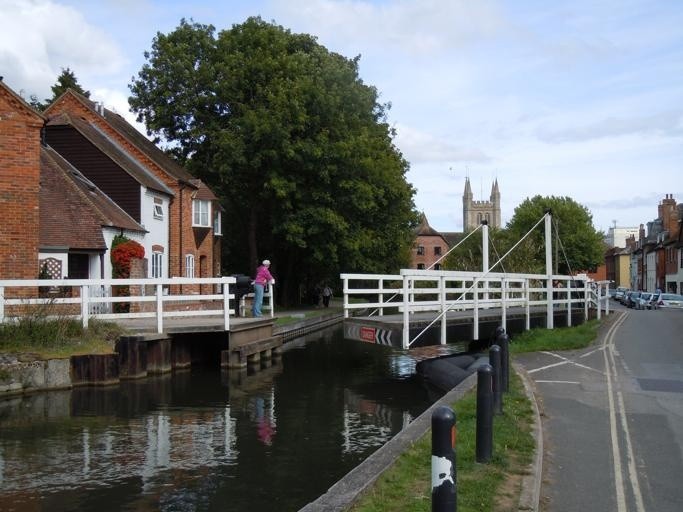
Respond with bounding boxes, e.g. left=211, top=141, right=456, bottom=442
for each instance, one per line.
left=553, top=280, right=565, bottom=307
left=251, top=259, right=275, bottom=317
left=322, top=282, right=334, bottom=308
left=254, top=396, right=272, bottom=447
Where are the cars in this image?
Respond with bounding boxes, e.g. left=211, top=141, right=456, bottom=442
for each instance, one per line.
left=595, top=286, right=683, bottom=310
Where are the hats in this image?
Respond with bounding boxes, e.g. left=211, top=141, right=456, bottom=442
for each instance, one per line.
left=262, top=260, right=271, bottom=265
